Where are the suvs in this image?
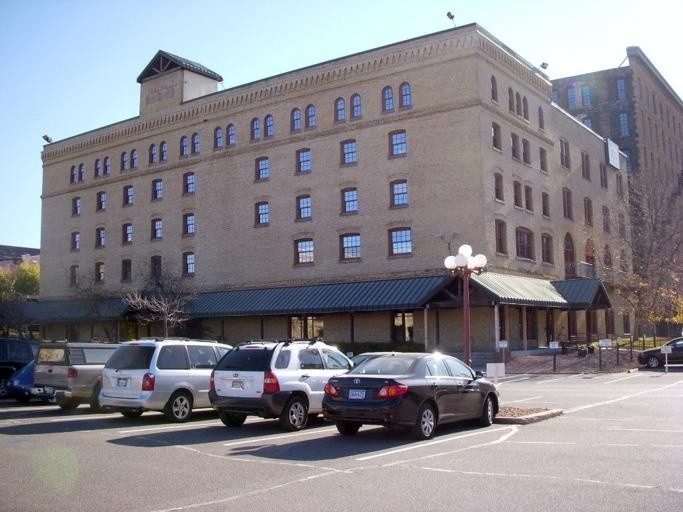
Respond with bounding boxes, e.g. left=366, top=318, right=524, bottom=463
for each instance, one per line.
left=206, top=338, right=354, bottom=431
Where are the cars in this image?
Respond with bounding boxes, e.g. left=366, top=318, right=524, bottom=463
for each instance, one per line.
left=6, top=358, right=59, bottom=405
left=349, top=351, right=391, bottom=366
left=321, top=352, right=500, bottom=438
left=636, top=337, right=682, bottom=368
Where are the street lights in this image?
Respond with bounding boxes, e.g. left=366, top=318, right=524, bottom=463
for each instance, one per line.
left=442, top=244, right=488, bottom=365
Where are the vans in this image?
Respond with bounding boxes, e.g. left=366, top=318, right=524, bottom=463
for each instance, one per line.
left=0, top=337, right=45, bottom=397
left=98, top=335, right=234, bottom=421
left=32, top=339, right=120, bottom=413
